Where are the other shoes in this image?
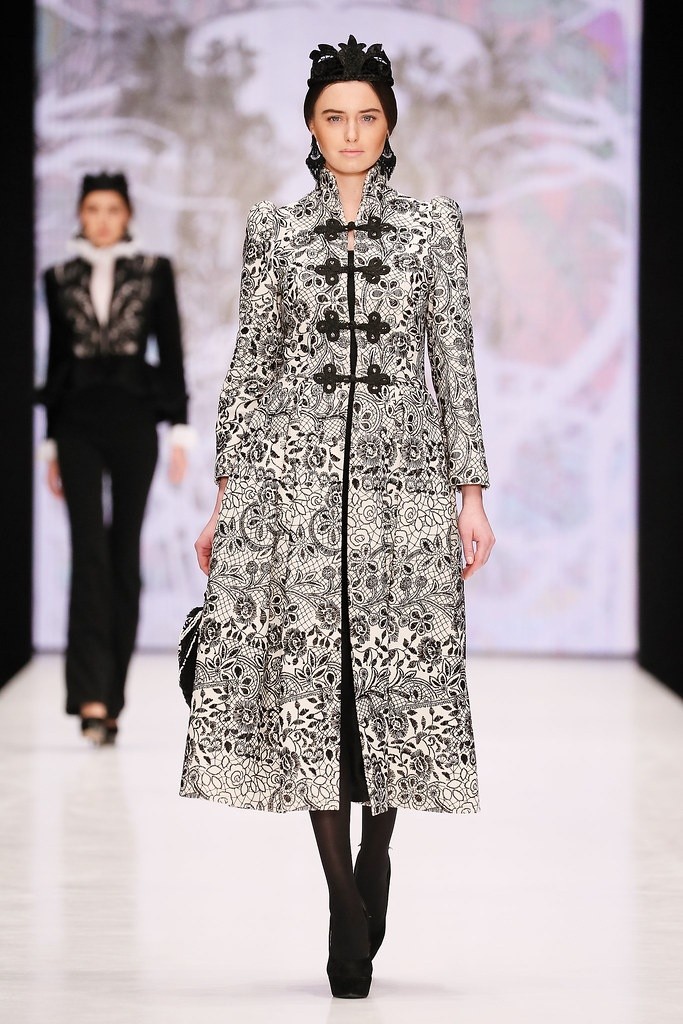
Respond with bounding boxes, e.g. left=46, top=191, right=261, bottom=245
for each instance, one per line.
left=326, top=899, right=372, bottom=998
left=353, top=843, right=390, bottom=960
left=80, top=711, right=117, bottom=747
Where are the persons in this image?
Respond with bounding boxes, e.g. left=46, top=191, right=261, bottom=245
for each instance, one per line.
left=42, top=173, right=190, bottom=745
left=178, top=34, right=496, bottom=998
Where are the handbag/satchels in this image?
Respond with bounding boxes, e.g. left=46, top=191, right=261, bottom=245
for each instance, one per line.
left=178, top=606, right=205, bottom=709
